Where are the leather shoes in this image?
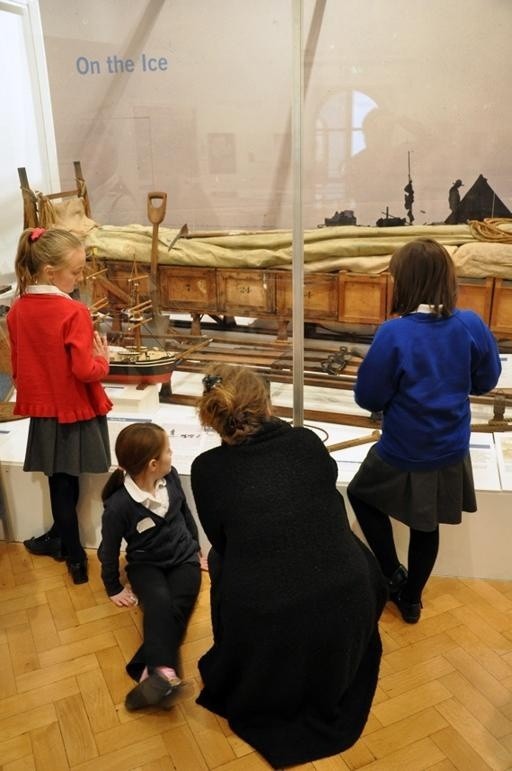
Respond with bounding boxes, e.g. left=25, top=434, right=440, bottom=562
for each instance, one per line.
left=69, top=561, right=89, bottom=585
left=390, top=593, right=421, bottom=625
left=24, top=533, right=64, bottom=561
left=380, top=564, right=409, bottom=591
left=171, top=680, right=195, bottom=700
left=126, top=675, right=172, bottom=712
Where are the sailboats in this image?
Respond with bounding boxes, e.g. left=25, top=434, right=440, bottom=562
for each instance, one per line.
left=85, top=251, right=214, bottom=385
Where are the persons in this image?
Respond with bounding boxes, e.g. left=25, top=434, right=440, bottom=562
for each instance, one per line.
left=190, top=361, right=390, bottom=770
left=97, top=423, right=209, bottom=710
left=346, top=237, right=501, bottom=624
left=6, top=227, right=113, bottom=585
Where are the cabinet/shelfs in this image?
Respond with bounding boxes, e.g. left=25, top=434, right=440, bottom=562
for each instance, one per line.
left=80, top=258, right=511, bottom=344
